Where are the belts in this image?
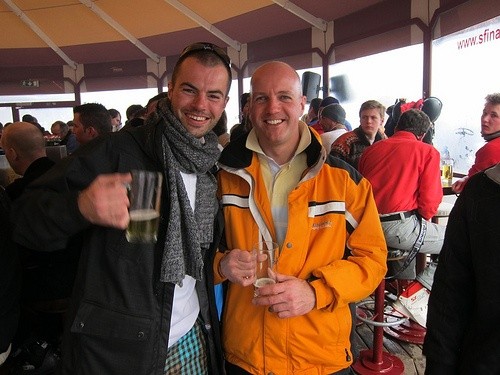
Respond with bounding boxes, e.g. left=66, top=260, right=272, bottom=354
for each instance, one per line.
left=380, top=210, right=417, bottom=222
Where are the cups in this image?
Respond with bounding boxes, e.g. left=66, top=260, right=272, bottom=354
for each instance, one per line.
left=442, top=159, right=453, bottom=188
left=115, top=170, right=163, bottom=243
left=250, top=241, right=279, bottom=300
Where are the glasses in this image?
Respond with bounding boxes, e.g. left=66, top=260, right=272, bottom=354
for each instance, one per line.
left=179, top=44, right=232, bottom=67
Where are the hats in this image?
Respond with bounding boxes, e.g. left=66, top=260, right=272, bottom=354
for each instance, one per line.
left=322, top=103, right=345, bottom=124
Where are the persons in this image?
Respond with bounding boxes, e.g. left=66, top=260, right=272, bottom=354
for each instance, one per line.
left=451, top=93, right=500, bottom=194
left=0, top=42, right=232, bottom=374
left=213, top=62, right=386, bottom=374
left=421, top=163, right=500, bottom=375
left=360, top=109, right=446, bottom=328
left=330, top=95, right=385, bottom=172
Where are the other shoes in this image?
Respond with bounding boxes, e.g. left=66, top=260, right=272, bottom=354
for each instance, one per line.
left=392, top=287, right=430, bottom=328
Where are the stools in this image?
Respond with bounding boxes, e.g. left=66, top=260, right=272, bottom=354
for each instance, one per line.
left=350, top=202, right=455, bottom=375
